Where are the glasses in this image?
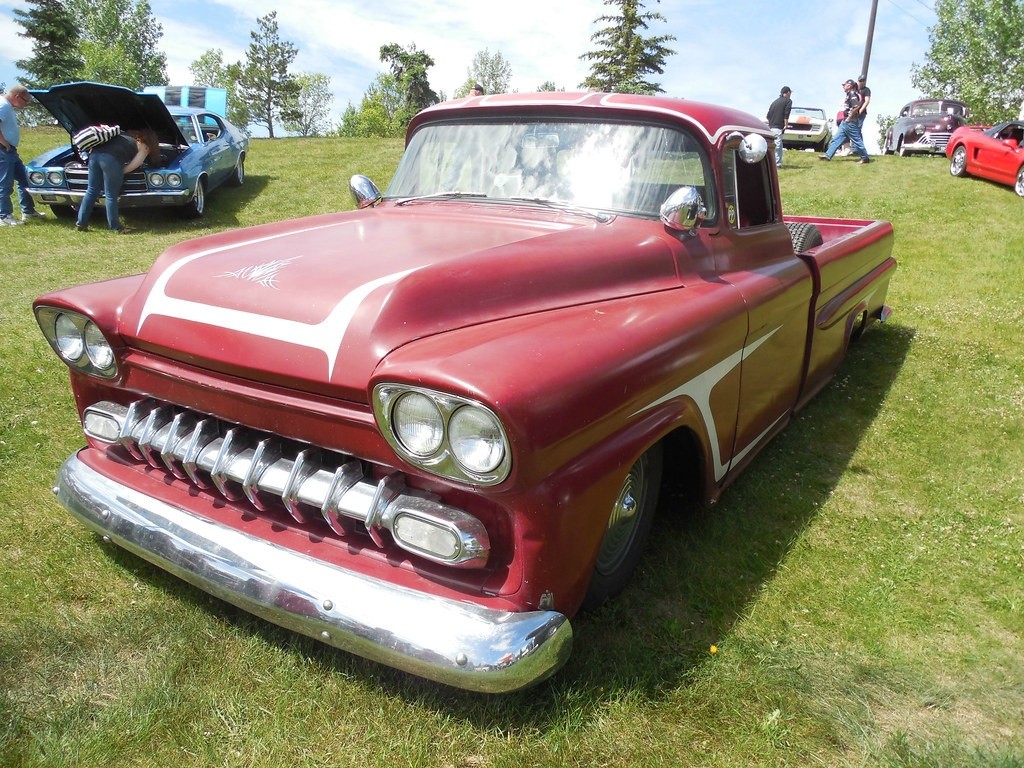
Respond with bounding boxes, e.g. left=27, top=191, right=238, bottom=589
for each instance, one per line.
left=15, top=94, right=29, bottom=102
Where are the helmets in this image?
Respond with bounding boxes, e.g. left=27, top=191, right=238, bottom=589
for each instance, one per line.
left=857, top=74, right=866, bottom=80
left=842, top=80, right=855, bottom=87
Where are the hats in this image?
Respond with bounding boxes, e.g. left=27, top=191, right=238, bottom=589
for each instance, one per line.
left=781, top=86, right=793, bottom=93
left=470, top=85, right=483, bottom=90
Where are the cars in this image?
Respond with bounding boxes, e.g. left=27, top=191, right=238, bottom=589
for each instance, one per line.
left=781, top=106, right=835, bottom=153
left=883, top=100, right=968, bottom=157
left=24, top=81, right=250, bottom=218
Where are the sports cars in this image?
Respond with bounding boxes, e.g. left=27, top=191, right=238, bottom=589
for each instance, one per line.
left=945, top=122, right=1024, bottom=197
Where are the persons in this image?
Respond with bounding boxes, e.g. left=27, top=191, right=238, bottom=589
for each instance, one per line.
left=818, top=73, right=871, bottom=163
left=77, top=126, right=158, bottom=234
left=767, top=87, right=792, bottom=167
left=0, top=86, right=46, bottom=226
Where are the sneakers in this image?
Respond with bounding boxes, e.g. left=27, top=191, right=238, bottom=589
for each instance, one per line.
left=0, top=214, right=25, bottom=226
left=22, top=209, right=46, bottom=220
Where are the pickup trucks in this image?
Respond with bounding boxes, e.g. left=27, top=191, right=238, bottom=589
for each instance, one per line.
left=32, top=84, right=899, bottom=695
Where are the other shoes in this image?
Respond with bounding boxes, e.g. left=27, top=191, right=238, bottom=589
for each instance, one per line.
left=857, top=158, right=869, bottom=164
left=115, top=228, right=131, bottom=234
left=75, top=221, right=87, bottom=228
left=818, top=155, right=830, bottom=161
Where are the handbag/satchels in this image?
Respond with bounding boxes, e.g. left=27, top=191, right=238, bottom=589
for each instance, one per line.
left=73, top=123, right=121, bottom=152
